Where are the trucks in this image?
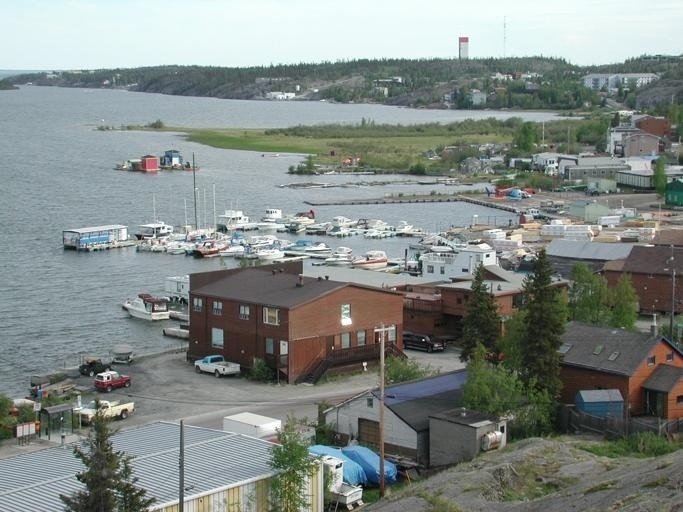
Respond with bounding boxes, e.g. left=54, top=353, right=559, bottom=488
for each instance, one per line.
left=512, top=189, right=522, bottom=199
left=521, top=192, right=532, bottom=199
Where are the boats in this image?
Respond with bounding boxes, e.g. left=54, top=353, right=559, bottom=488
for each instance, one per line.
left=514, top=207, right=546, bottom=219
left=120, top=293, right=170, bottom=321
left=58, top=222, right=135, bottom=253
left=112, top=154, right=161, bottom=172
left=159, top=147, right=199, bottom=172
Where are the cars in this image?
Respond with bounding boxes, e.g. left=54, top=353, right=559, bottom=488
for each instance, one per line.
left=470, top=349, right=502, bottom=362
left=341, top=445, right=399, bottom=486
left=79, top=398, right=136, bottom=427
left=78, top=357, right=112, bottom=376
left=310, top=444, right=367, bottom=488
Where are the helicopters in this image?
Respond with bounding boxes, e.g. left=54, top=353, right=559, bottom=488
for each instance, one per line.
left=485, top=183, right=537, bottom=200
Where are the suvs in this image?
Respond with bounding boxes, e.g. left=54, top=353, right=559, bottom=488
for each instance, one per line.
left=402, top=330, right=447, bottom=354
left=96, top=371, right=131, bottom=392
left=584, top=188, right=599, bottom=196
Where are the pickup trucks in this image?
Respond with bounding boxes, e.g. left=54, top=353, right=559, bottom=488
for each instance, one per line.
left=193, top=354, right=241, bottom=378
